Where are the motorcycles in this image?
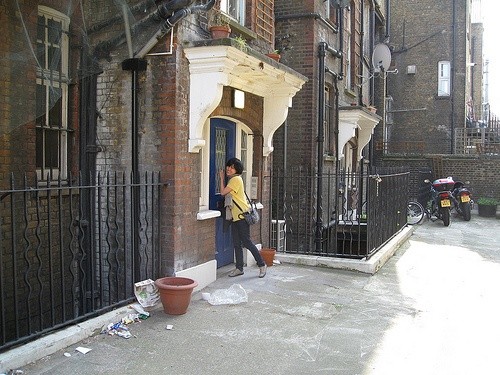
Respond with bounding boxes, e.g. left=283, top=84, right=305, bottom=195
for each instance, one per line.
left=424, top=176, right=474, bottom=226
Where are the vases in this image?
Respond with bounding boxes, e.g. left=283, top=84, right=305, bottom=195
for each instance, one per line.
left=259, top=249, right=276, bottom=266
left=154, top=277, right=198, bottom=314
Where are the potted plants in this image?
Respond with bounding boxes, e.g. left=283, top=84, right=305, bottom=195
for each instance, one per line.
left=264, top=49, right=281, bottom=63
left=209, top=12, right=231, bottom=38
left=476, top=197, right=497, bottom=217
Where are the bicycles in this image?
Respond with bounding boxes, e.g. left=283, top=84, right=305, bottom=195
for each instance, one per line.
left=407, top=200, right=425, bottom=224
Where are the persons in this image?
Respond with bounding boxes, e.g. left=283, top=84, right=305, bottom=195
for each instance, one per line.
left=219, top=158, right=268, bottom=278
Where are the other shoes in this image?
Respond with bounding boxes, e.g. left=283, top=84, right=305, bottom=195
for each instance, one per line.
left=258, top=264, right=267, bottom=278
left=229, top=269, right=243, bottom=276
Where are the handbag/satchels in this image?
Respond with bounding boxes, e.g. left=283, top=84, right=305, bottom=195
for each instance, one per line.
left=242, top=206, right=260, bottom=225
